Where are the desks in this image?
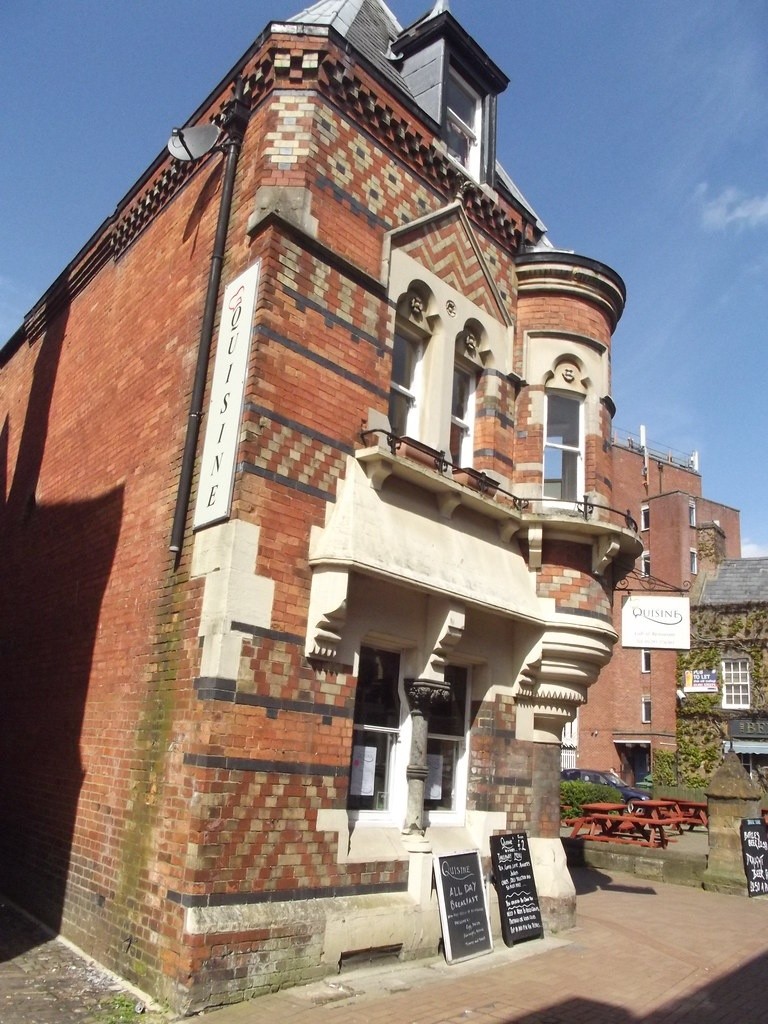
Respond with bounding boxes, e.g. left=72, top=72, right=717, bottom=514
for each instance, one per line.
left=677, top=802, right=707, bottom=831
left=630, top=800, right=683, bottom=849
left=582, top=803, right=627, bottom=837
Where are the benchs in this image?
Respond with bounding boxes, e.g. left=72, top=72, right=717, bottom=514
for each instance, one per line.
left=564, top=811, right=707, bottom=850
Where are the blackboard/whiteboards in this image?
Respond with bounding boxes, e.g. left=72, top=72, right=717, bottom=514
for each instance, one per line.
left=740, top=818, right=768, bottom=897
left=490, top=834, right=544, bottom=941
left=434, top=850, right=494, bottom=965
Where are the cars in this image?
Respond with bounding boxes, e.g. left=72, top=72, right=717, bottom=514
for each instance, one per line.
left=559, top=767, right=653, bottom=815
